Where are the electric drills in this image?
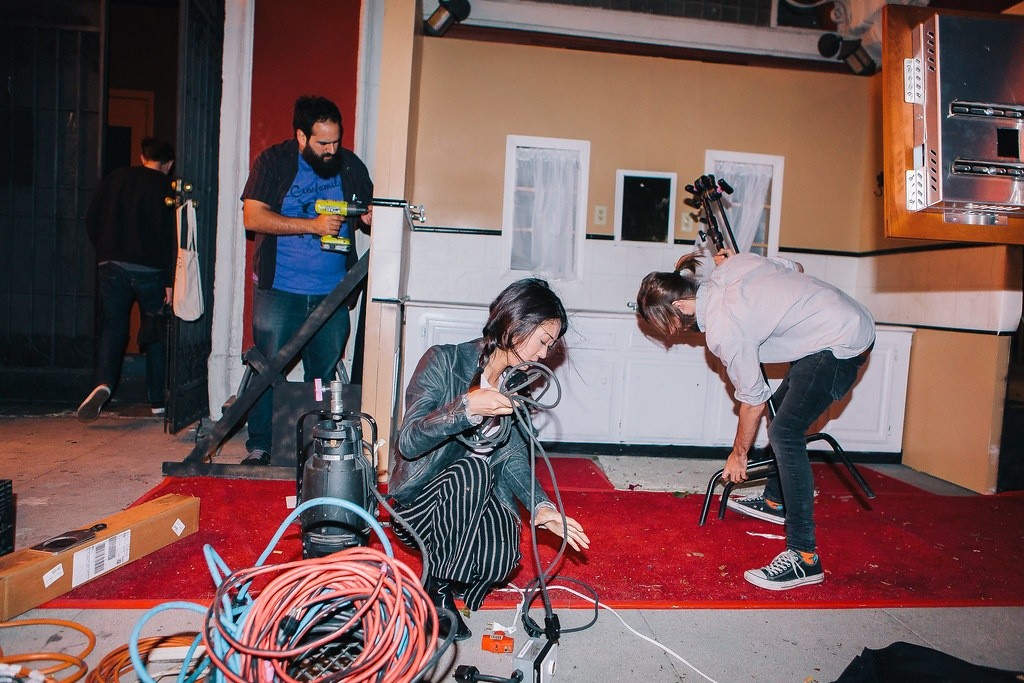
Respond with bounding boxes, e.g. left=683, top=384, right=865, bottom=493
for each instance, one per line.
left=315, top=199, right=373, bottom=252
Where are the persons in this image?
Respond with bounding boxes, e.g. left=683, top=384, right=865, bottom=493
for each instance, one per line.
left=388, top=277, right=590, bottom=643
left=636, top=250, right=876, bottom=591
left=77, top=134, right=176, bottom=424
left=239, top=93, right=375, bottom=472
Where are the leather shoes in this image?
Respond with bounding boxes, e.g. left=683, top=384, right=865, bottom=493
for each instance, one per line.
left=423, top=575, right=472, bottom=641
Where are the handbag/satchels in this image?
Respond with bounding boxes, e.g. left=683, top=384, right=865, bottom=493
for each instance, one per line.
left=173, top=198, right=204, bottom=322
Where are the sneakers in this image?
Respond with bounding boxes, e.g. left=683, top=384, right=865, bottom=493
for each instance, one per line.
left=743, top=548, right=825, bottom=592
left=719, top=492, right=788, bottom=525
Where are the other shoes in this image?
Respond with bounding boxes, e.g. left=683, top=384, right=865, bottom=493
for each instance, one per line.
left=150, top=400, right=165, bottom=414
left=240, top=449, right=271, bottom=469
left=75, top=382, right=112, bottom=423
